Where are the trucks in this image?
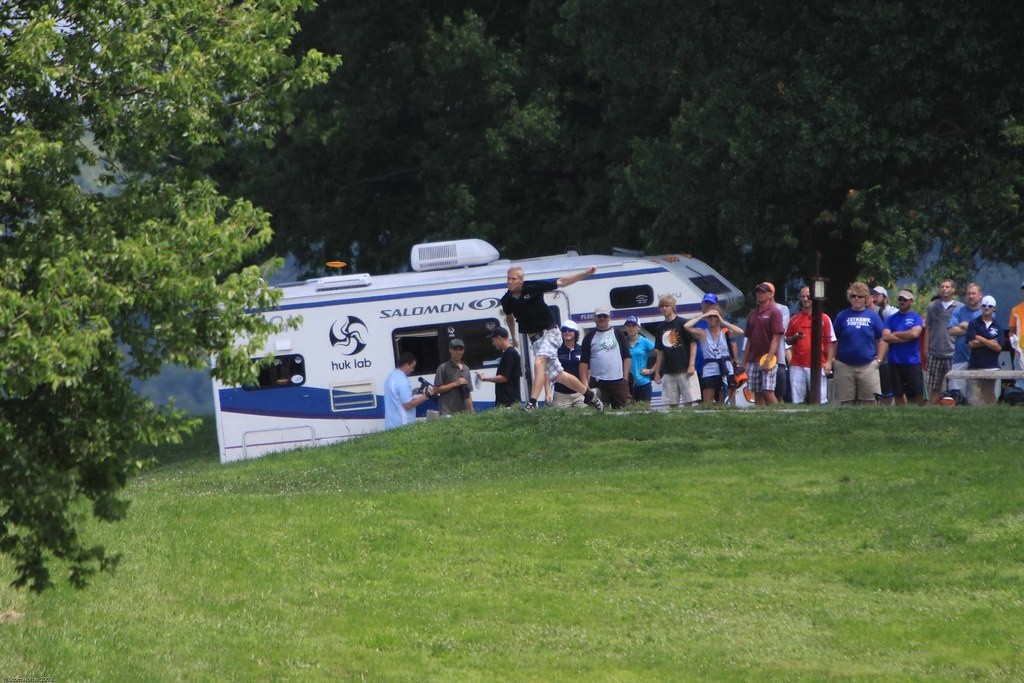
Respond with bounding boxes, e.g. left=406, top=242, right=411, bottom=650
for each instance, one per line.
left=209, top=238, right=744, bottom=461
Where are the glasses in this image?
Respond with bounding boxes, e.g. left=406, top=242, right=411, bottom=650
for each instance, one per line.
left=982, top=304, right=993, bottom=308
left=850, top=294, right=866, bottom=298
left=898, top=296, right=908, bottom=302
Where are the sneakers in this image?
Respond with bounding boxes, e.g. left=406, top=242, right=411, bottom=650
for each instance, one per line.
left=520, top=403, right=535, bottom=414
left=588, top=388, right=603, bottom=414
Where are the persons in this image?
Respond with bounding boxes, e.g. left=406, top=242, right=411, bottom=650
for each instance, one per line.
left=435, top=339, right=476, bottom=419
left=549, top=277, right=1024, bottom=411
left=474, top=327, right=522, bottom=408
left=501, top=265, right=606, bottom=414
left=386, top=352, right=433, bottom=432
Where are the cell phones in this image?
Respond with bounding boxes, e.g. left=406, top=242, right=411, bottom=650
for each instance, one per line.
left=798, top=332, right=803, bottom=335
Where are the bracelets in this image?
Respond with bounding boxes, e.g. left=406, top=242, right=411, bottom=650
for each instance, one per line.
left=876, top=358, right=882, bottom=363
left=650, top=369, right=652, bottom=374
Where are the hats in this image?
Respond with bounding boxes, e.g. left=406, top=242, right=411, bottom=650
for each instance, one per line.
left=594, top=306, right=610, bottom=316
left=703, top=293, right=718, bottom=304
left=982, top=295, right=996, bottom=306
left=449, top=339, right=464, bottom=348
left=486, top=327, right=508, bottom=339
left=898, top=290, right=914, bottom=299
left=625, top=316, right=640, bottom=325
left=560, top=320, right=578, bottom=331
left=755, top=282, right=775, bottom=292
left=873, top=286, right=887, bottom=295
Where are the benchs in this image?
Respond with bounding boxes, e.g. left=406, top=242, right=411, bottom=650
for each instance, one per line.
left=944, top=370, right=1024, bottom=405
